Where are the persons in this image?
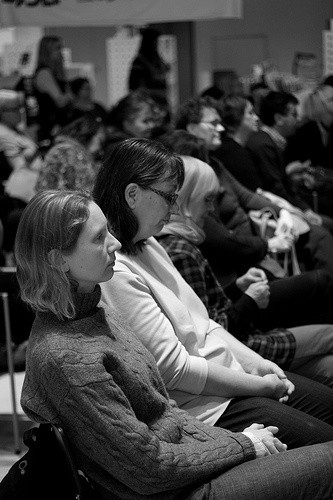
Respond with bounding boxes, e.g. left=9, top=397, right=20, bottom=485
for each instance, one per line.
left=0, top=27, right=333, bottom=451
left=15, top=191, right=333, bottom=500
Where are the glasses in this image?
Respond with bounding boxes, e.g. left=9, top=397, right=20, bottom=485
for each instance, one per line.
left=148, top=186, right=179, bottom=206
left=199, top=120, right=222, bottom=126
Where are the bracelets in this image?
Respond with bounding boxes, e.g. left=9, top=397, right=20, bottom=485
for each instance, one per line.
left=271, top=238, right=277, bottom=253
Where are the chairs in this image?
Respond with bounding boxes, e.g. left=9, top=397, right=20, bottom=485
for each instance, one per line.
left=24, top=422, right=83, bottom=500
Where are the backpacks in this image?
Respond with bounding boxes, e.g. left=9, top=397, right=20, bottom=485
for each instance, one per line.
left=0, top=423, right=79, bottom=500
